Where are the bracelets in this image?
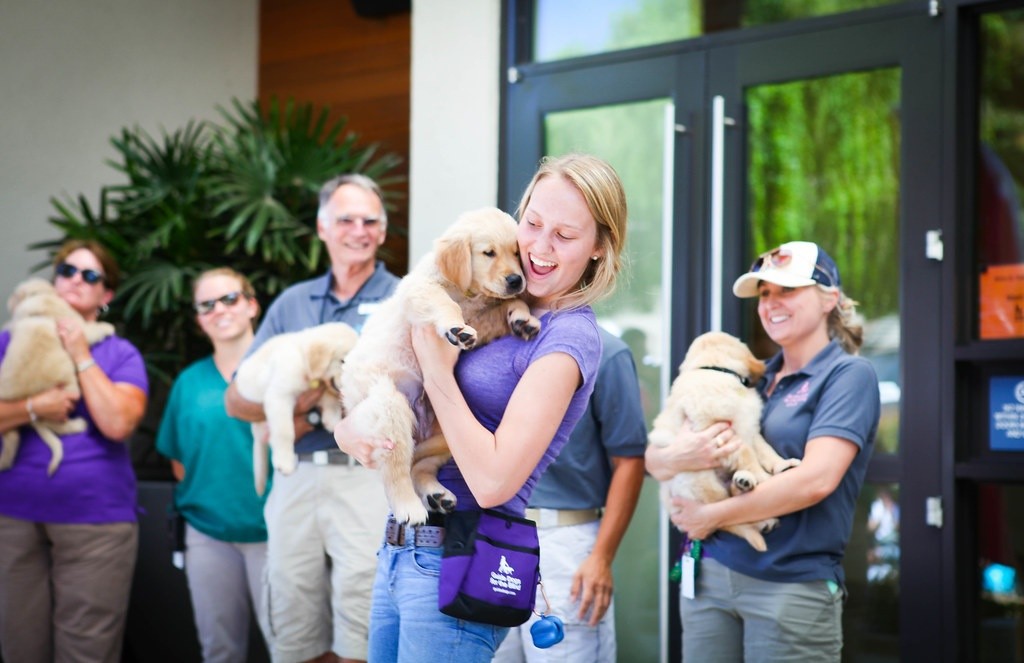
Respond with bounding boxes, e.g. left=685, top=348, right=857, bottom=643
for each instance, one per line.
left=75, top=358, right=96, bottom=372
left=27, top=398, right=39, bottom=422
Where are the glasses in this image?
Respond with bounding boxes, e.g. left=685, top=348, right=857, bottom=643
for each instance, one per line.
left=335, top=217, right=380, bottom=230
left=749, top=250, right=791, bottom=272
left=56, top=263, right=107, bottom=286
left=195, top=292, right=248, bottom=315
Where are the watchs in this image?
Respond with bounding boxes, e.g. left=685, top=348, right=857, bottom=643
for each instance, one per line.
left=307, top=408, right=323, bottom=432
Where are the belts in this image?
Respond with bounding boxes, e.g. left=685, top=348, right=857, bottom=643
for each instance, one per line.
left=296, top=448, right=360, bottom=465
left=519, top=507, right=599, bottom=528
left=385, top=514, right=445, bottom=548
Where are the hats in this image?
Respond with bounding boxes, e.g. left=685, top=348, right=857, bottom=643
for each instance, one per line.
left=732, top=241, right=839, bottom=298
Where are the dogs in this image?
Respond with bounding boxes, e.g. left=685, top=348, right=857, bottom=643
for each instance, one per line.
left=0, top=276, right=117, bottom=476
left=336, top=205, right=542, bottom=528
left=646, top=331, right=800, bottom=554
left=235, top=319, right=360, bottom=499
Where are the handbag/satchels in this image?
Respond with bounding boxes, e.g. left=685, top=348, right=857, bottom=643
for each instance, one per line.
left=438, top=509, right=540, bottom=627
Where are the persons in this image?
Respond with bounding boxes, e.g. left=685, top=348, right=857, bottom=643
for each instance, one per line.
left=226, top=175, right=403, bottom=663
left=644, top=242, right=880, bottom=663
left=491, top=325, right=648, bottom=663
left=366, top=154, right=629, bottom=663
left=0, top=242, right=147, bottom=663
left=157, top=268, right=273, bottom=663
